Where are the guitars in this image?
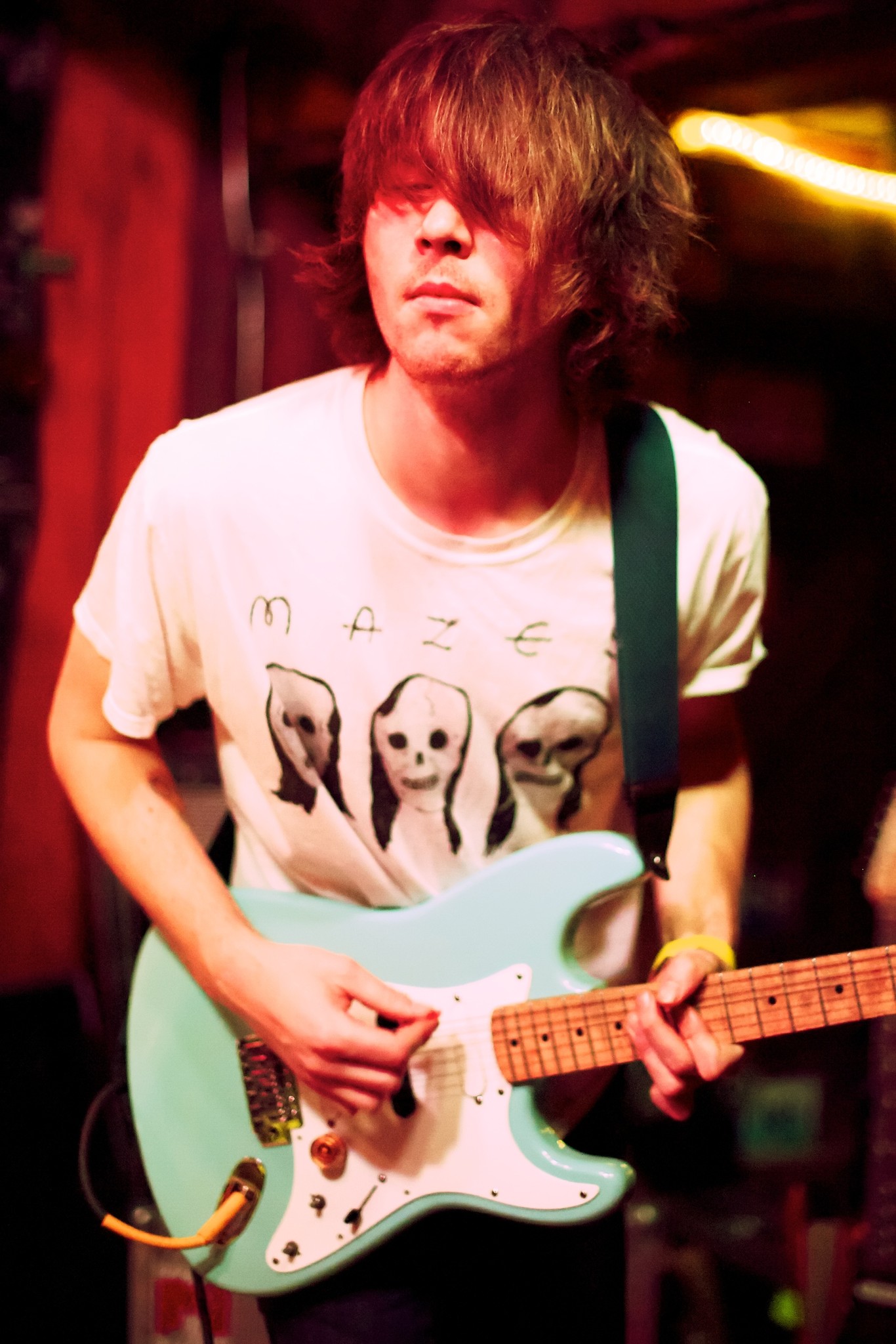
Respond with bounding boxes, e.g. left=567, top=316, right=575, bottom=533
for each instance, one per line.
left=126, top=829, right=896, bottom=1296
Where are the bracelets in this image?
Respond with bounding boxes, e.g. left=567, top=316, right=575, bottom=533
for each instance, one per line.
left=647, top=934, right=736, bottom=982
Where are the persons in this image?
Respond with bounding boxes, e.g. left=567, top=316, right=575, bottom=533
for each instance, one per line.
left=46, top=15, right=770, bottom=1344
